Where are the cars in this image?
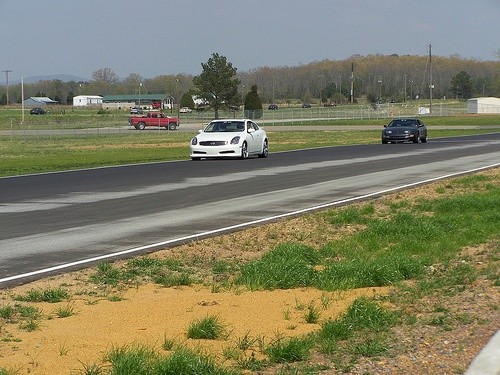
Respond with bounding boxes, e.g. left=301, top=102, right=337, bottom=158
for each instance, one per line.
left=30, top=108, right=46, bottom=114
left=268, top=105, right=278, bottom=110
left=382, top=119, right=428, bottom=144
left=302, top=102, right=312, bottom=108
left=323, top=101, right=336, bottom=107
left=180, top=106, right=193, bottom=113
left=189, top=118, right=269, bottom=161
left=152, top=102, right=161, bottom=108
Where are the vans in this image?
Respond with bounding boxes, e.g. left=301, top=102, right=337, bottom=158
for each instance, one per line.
left=130, top=106, right=143, bottom=114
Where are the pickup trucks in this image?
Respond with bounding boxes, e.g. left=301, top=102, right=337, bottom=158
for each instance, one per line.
left=127, top=111, right=180, bottom=130
